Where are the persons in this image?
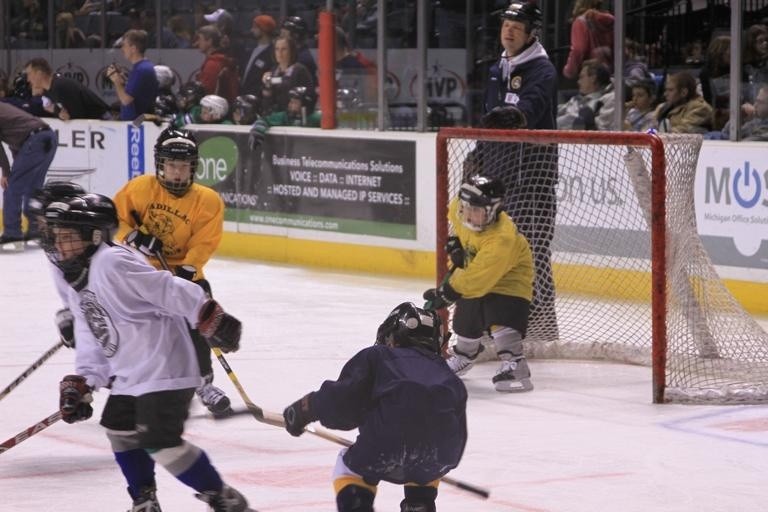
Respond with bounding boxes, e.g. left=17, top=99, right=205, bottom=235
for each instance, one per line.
left=44, top=192, right=249, bottom=512
left=621, top=38, right=649, bottom=81
left=23, top=56, right=115, bottom=120
left=623, top=82, right=660, bottom=132
left=85, top=34, right=107, bottom=50
left=106, top=28, right=159, bottom=120
left=282, top=15, right=318, bottom=84
left=112, top=127, right=226, bottom=418
left=195, top=24, right=240, bottom=95
left=462, top=0, right=561, bottom=342
left=722, top=83, right=768, bottom=142
left=699, top=37, right=732, bottom=105
left=237, top=14, right=277, bottom=97
left=247, top=85, right=322, bottom=154
left=422, top=170, right=535, bottom=395
left=260, top=37, right=319, bottom=118
left=246, top=117, right=247, bottom=119
left=555, top=58, right=617, bottom=130
left=229, top=95, right=258, bottom=125
left=332, top=25, right=369, bottom=113
left=197, top=93, right=230, bottom=125
left=655, top=72, right=716, bottom=135
left=165, top=83, right=203, bottom=126
left=0, top=101, right=58, bottom=241
left=562, top=0, right=615, bottom=80
left=283, top=301, right=468, bottom=512
left=54, top=11, right=87, bottom=51
left=742, top=24, right=768, bottom=82
left=201, top=8, right=235, bottom=42
left=24, top=179, right=86, bottom=350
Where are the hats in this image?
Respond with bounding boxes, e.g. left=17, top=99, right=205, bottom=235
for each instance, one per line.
left=281, top=14, right=303, bottom=34
left=154, top=63, right=174, bottom=90
left=204, top=9, right=232, bottom=31
left=233, top=94, right=258, bottom=111
left=254, top=14, right=276, bottom=32
left=200, top=95, right=230, bottom=116
left=287, top=86, right=316, bottom=106
left=177, top=81, right=203, bottom=97
left=313, top=24, right=348, bottom=48
left=494, top=1, right=544, bottom=25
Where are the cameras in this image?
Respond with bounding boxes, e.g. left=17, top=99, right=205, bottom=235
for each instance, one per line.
left=101, top=63, right=123, bottom=90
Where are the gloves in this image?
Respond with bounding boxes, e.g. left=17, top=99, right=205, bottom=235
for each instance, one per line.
left=126, top=230, right=163, bottom=258
left=55, top=308, right=77, bottom=349
left=196, top=298, right=243, bottom=354
left=444, top=236, right=465, bottom=268
left=421, top=280, right=462, bottom=312
left=59, top=374, right=94, bottom=424
left=282, top=391, right=317, bottom=439
left=172, top=264, right=196, bottom=283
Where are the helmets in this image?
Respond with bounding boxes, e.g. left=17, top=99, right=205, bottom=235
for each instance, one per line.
left=456, top=174, right=504, bottom=232
left=153, top=126, right=200, bottom=196
left=374, top=302, right=441, bottom=354
left=24, top=181, right=85, bottom=240
left=46, top=193, right=119, bottom=274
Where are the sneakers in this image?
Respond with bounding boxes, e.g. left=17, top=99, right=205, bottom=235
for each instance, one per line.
left=444, top=354, right=476, bottom=375
left=197, top=375, right=230, bottom=413
left=25, top=229, right=47, bottom=248
left=490, top=350, right=531, bottom=384
left=0, top=233, right=25, bottom=251
left=196, top=485, right=261, bottom=512
left=123, top=487, right=161, bottom=511
